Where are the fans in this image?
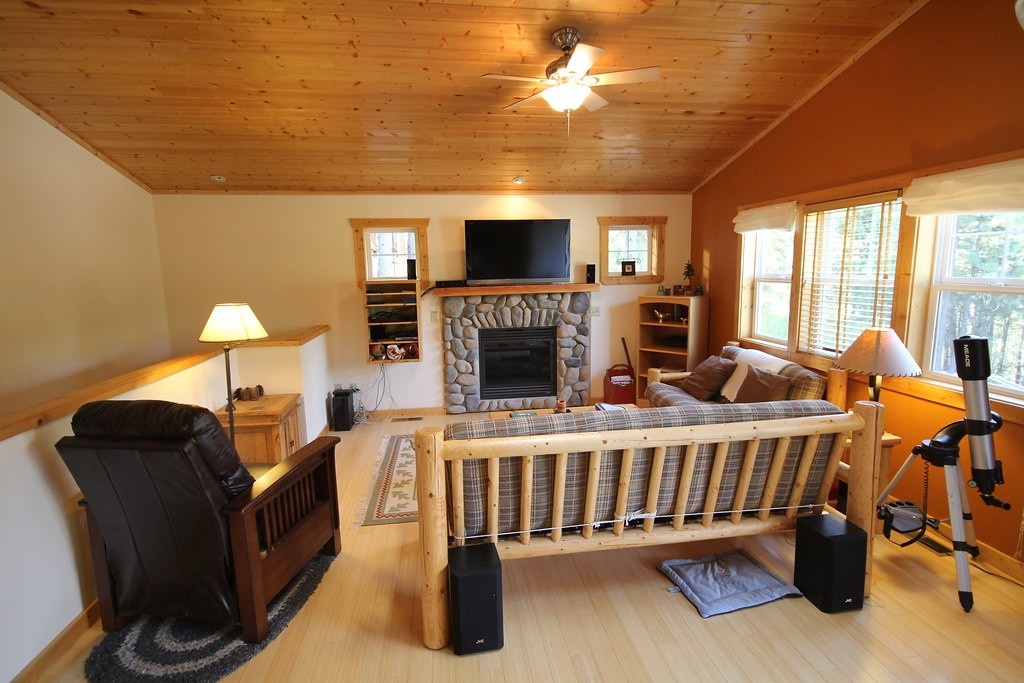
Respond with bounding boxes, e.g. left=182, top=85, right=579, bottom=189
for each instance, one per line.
left=481, top=27, right=661, bottom=112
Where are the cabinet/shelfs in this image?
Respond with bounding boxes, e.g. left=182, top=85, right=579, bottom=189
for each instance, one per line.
left=212, top=392, right=302, bottom=473
left=635, top=295, right=711, bottom=407
left=363, top=280, right=422, bottom=363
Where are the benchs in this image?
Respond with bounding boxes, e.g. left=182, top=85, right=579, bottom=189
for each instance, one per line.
left=413, top=342, right=848, bottom=650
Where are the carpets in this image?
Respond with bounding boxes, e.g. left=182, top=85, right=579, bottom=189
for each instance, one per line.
left=352, top=435, right=418, bottom=526
left=84, top=550, right=338, bottom=683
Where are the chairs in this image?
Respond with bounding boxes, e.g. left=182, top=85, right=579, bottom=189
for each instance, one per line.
left=54, top=401, right=341, bottom=642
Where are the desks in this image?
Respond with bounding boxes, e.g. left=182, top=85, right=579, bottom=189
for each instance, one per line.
left=845, top=427, right=902, bottom=534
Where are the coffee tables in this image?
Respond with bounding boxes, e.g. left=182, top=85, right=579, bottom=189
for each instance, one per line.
left=489, top=403, right=638, bottom=421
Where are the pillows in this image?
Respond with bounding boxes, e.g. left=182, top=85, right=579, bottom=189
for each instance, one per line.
left=733, top=365, right=792, bottom=404
left=680, top=356, right=737, bottom=402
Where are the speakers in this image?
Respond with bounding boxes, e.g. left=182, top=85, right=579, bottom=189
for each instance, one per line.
left=586, top=264, right=595, bottom=284
left=407, top=258, right=417, bottom=280
left=447, top=541, right=504, bottom=658
left=794, top=514, right=868, bottom=616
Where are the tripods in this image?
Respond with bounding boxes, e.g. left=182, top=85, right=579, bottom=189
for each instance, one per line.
left=877, top=440, right=981, bottom=612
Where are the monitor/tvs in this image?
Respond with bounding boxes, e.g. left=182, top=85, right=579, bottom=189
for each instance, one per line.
left=465, top=219, right=571, bottom=285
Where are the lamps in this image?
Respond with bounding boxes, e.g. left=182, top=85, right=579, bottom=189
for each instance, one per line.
left=833, top=326, right=924, bottom=401
left=199, top=302, right=270, bottom=447
left=540, top=82, right=591, bottom=136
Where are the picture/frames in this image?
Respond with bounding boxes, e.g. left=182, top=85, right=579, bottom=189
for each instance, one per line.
left=622, top=261, right=635, bottom=276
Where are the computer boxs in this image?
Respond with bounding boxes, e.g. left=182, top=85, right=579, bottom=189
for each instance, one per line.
left=333, top=389, right=355, bottom=431
